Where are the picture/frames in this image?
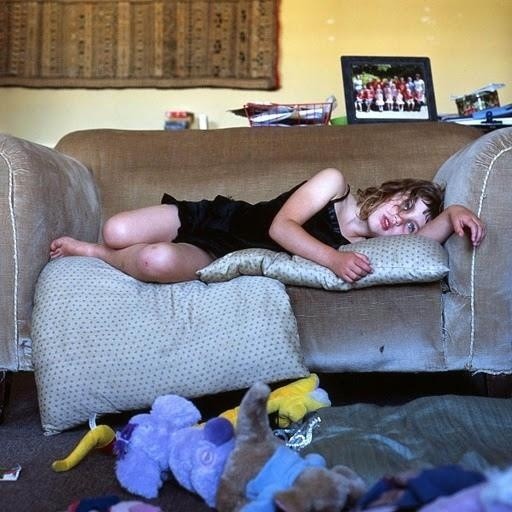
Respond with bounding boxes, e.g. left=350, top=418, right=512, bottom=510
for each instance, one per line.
left=341, top=55, right=438, bottom=123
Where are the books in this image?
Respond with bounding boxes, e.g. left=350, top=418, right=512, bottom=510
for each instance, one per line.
left=165, top=111, right=196, bottom=131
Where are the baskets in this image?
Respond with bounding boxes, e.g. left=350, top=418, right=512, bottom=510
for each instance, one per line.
left=245, top=99, right=333, bottom=127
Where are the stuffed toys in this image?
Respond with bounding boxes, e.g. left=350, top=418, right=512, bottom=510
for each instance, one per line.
left=48, top=371, right=512, bottom=512
left=47, top=373, right=512, bottom=512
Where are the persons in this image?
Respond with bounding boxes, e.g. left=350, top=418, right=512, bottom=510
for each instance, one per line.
left=49, top=167, right=487, bottom=285
left=352, top=74, right=429, bottom=113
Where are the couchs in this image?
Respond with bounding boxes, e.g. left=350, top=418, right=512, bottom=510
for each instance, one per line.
left=0, top=120, right=512, bottom=423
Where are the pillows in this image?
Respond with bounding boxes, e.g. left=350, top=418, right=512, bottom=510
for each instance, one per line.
left=198, top=227, right=450, bottom=291
left=31, top=249, right=311, bottom=436
